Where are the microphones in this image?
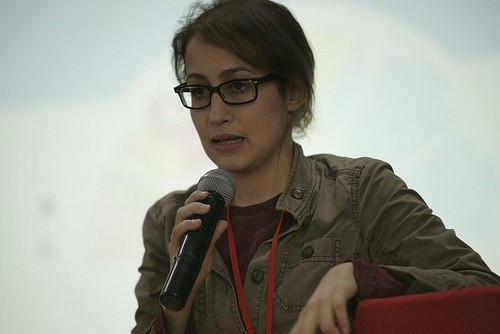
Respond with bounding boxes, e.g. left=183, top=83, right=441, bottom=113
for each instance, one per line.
left=158, top=169, right=237, bottom=313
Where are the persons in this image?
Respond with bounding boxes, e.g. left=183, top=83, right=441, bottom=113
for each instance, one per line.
left=130, top=0, right=499, bottom=334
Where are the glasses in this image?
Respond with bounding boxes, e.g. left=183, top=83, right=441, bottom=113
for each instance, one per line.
left=174, top=72, right=278, bottom=109
